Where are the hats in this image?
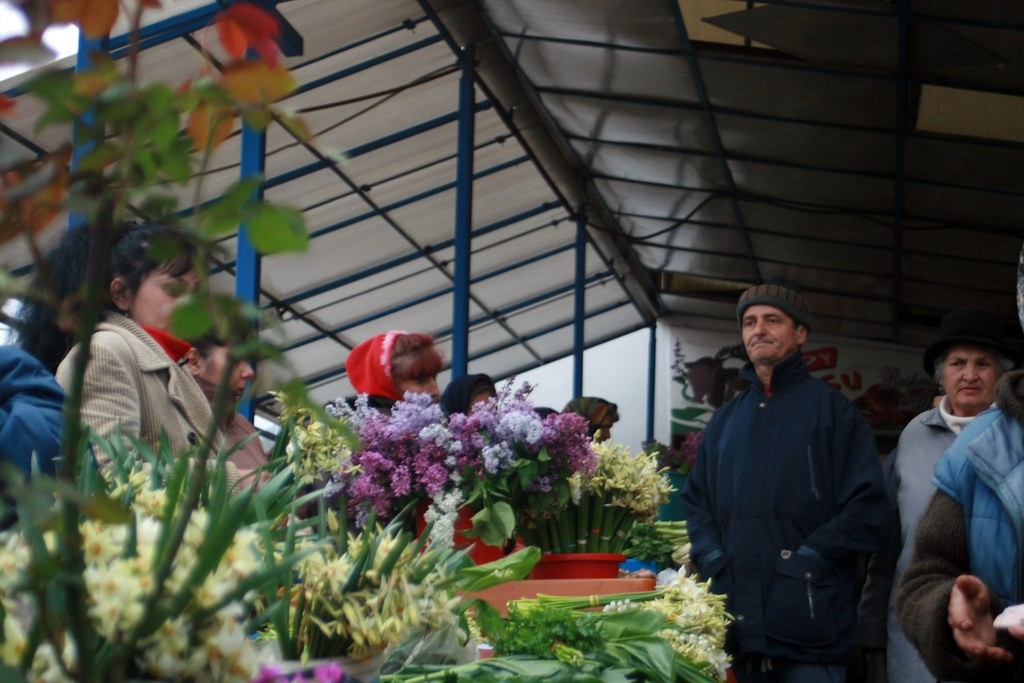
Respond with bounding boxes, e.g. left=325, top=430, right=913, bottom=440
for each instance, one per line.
left=736, top=277, right=811, bottom=333
left=923, top=307, right=1023, bottom=378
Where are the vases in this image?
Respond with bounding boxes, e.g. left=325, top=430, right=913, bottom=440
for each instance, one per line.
left=527, top=554, right=626, bottom=579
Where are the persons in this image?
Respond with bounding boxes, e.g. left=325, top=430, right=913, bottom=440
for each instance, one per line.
left=559, top=397, right=618, bottom=447
left=1, top=270, right=69, bottom=533
left=440, top=373, right=498, bottom=427
left=679, top=276, right=890, bottom=683
left=171, top=294, right=319, bottom=535
left=898, top=252, right=1024, bottom=683
left=14, top=220, right=271, bottom=519
left=884, top=307, right=1014, bottom=681
left=327, top=332, right=445, bottom=418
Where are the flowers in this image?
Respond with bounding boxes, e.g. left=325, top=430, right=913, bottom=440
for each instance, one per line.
left=0, top=0, right=733, bottom=683
